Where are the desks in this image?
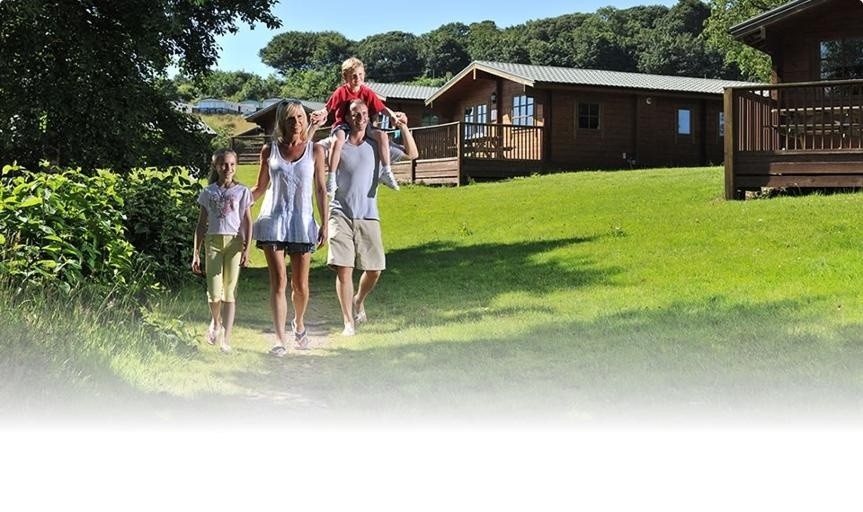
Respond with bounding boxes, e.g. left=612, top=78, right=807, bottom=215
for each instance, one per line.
left=464, top=136, right=504, bottom=158
left=771, top=104, right=863, bottom=148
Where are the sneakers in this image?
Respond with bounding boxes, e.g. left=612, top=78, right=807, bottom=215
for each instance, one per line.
left=326, top=180, right=338, bottom=194
left=380, top=171, right=400, bottom=191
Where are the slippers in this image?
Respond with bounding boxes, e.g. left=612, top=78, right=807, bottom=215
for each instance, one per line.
left=267, top=344, right=289, bottom=357
left=290, top=318, right=309, bottom=350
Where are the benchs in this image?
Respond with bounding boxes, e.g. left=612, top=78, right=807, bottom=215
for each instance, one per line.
left=448, top=146, right=513, bottom=159
left=763, top=122, right=862, bottom=149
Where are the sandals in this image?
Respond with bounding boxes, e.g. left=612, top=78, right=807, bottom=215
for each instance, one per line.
left=341, top=294, right=368, bottom=337
left=205, top=319, right=232, bottom=354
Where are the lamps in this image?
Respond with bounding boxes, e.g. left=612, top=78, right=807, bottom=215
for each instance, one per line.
left=491, top=91, right=497, bottom=104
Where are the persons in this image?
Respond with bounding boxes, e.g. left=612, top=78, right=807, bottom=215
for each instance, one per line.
left=307, top=97, right=420, bottom=338
left=248, top=100, right=329, bottom=358
left=311, top=56, right=408, bottom=194
left=190, top=147, right=254, bottom=355
